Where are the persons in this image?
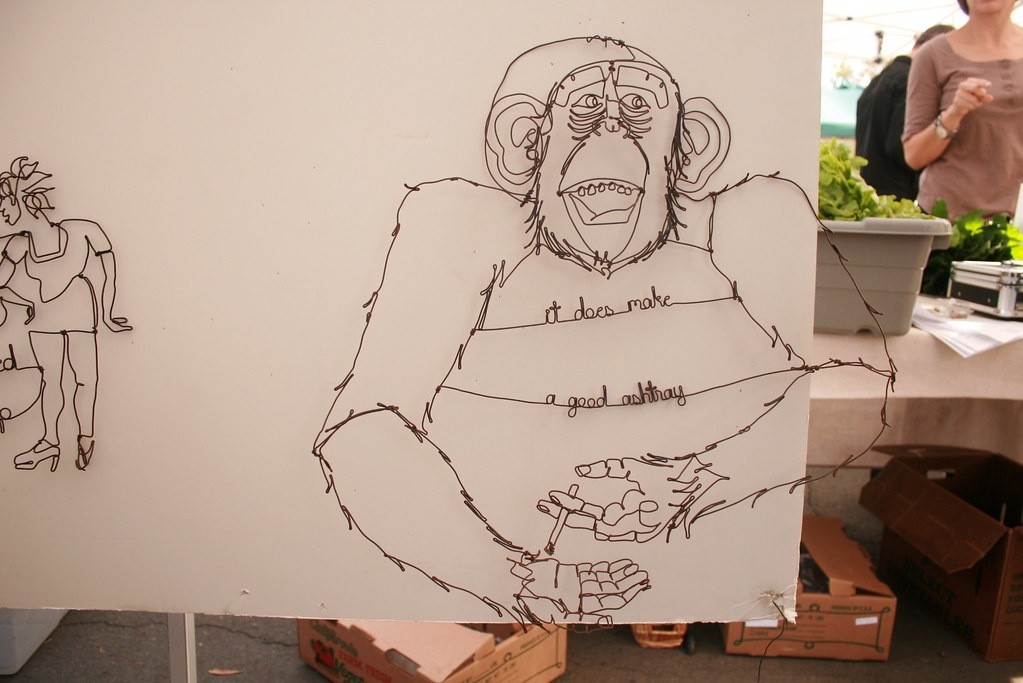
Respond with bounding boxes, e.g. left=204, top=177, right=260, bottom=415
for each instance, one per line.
left=855, top=24, right=955, bottom=201
left=898, top=0, right=1023, bottom=223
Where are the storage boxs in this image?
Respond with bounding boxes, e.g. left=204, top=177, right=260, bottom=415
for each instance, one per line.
left=718, top=513, right=897, bottom=662
left=860, top=444, right=1023, bottom=663
left=814, top=214, right=953, bottom=337
left=295, top=618, right=568, bottom=683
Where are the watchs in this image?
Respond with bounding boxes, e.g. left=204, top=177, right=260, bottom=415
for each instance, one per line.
left=933, top=111, right=958, bottom=139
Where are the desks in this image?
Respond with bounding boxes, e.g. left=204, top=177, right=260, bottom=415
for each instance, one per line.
left=807, top=295, right=1022, bottom=467
